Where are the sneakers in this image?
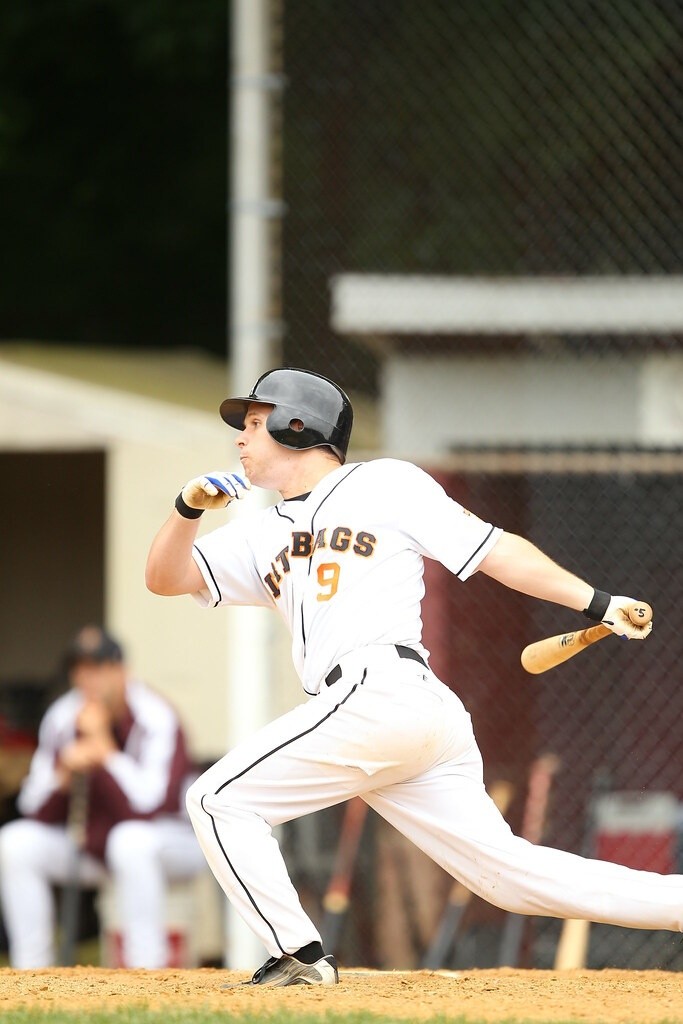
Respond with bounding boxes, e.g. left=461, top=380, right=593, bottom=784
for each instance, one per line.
left=219, top=955, right=338, bottom=987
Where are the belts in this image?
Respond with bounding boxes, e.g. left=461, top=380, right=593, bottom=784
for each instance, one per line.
left=325, top=645, right=429, bottom=687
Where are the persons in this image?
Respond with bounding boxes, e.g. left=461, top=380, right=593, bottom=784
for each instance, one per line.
left=0, top=623, right=206, bottom=971
left=144, top=369, right=683, bottom=989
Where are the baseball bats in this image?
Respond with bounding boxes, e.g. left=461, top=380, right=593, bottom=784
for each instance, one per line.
left=58, top=736, right=96, bottom=965
left=312, top=751, right=611, bottom=969
left=521, top=603, right=651, bottom=675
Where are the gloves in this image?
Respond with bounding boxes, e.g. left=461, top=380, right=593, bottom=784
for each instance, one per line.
left=174, top=470, right=251, bottom=520
left=583, top=586, right=652, bottom=640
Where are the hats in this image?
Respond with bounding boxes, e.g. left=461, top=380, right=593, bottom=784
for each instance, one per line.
left=61, top=624, right=121, bottom=667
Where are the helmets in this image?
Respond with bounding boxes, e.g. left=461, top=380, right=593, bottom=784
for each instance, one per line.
left=218, top=367, right=352, bottom=465
